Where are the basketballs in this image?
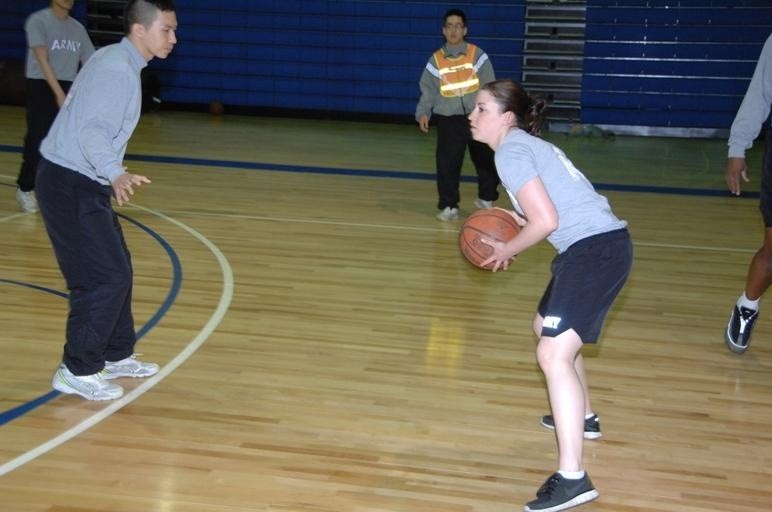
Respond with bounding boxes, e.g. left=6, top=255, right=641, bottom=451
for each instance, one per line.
left=459, top=209, right=521, bottom=270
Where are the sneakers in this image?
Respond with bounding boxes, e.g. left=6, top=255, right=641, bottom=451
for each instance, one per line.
left=541, top=415, right=603, bottom=439
left=725, top=303, right=760, bottom=355
left=474, top=197, right=493, bottom=209
left=523, top=470, right=600, bottom=512
left=97, top=353, right=160, bottom=380
left=15, top=187, right=41, bottom=213
left=51, top=363, right=124, bottom=401
left=436, top=206, right=459, bottom=222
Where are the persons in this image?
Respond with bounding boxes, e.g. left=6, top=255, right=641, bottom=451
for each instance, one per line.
left=34, top=2, right=178, bottom=402
left=724, top=32, right=772, bottom=354
left=14, top=1, right=97, bottom=215
left=416, top=8, right=495, bottom=224
left=466, top=79, right=632, bottom=511
